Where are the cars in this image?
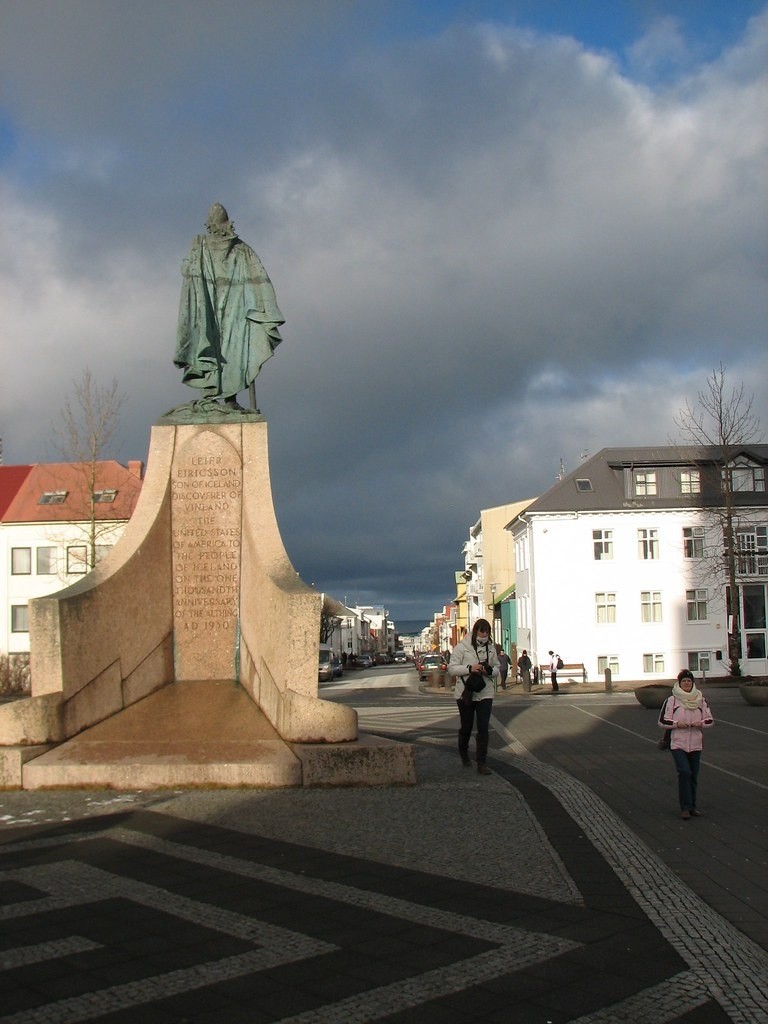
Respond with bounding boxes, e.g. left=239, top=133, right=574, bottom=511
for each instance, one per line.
left=333, top=658, right=343, bottom=677
left=419, top=655, right=447, bottom=681
left=373, top=654, right=393, bottom=664
left=356, top=656, right=372, bottom=668
left=412, top=655, right=426, bottom=670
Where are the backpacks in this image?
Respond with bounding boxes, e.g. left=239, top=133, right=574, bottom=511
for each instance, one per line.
left=557, top=657, right=564, bottom=669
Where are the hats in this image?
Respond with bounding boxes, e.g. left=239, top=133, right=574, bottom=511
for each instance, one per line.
left=523, top=650, right=526, bottom=654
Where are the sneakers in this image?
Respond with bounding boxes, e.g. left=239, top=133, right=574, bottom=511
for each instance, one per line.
left=681, top=809, right=690, bottom=819
left=476, top=764, right=492, bottom=774
left=459, top=750, right=472, bottom=766
left=690, top=810, right=701, bottom=816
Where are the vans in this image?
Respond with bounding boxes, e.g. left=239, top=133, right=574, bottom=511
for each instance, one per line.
left=395, top=652, right=406, bottom=663
left=319, top=643, right=334, bottom=681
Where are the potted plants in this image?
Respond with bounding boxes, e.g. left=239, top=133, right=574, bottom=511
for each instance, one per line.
left=636, top=684, right=674, bottom=709
left=739, top=680, right=768, bottom=706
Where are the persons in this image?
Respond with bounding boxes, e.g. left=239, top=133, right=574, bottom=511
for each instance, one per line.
left=549, top=650, right=561, bottom=691
left=444, top=650, right=450, bottom=664
left=497, top=650, right=512, bottom=690
left=658, top=669, right=713, bottom=819
left=517, top=650, right=533, bottom=685
left=171, top=206, right=287, bottom=413
left=449, top=620, right=501, bottom=776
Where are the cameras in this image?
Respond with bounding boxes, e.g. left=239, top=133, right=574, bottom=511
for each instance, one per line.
left=480, top=661, right=492, bottom=673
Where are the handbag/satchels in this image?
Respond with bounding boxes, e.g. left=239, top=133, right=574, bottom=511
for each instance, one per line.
left=657, top=728, right=671, bottom=751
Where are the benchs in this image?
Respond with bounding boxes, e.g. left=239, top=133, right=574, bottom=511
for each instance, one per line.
left=539, top=664, right=587, bottom=686
